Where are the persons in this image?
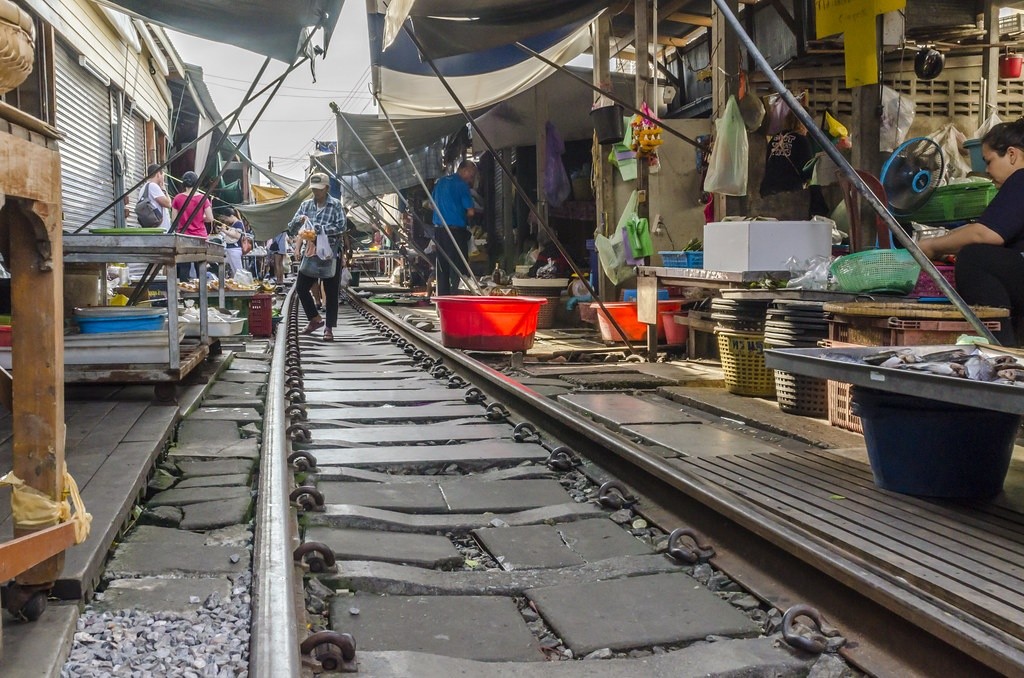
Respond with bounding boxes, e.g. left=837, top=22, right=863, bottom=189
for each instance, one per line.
left=288, top=173, right=345, bottom=341
left=524, top=227, right=575, bottom=278
left=172, top=171, right=213, bottom=283
left=431, top=160, right=477, bottom=296
left=271, top=233, right=289, bottom=284
left=222, top=208, right=245, bottom=278
left=138, top=164, right=171, bottom=276
left=916, top=116, right=1024, bottom=347
left=758, top=104, right=814, bottom=221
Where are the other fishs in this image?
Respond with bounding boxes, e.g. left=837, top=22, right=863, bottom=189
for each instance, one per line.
left=817, top=343, right=1024, bottom=384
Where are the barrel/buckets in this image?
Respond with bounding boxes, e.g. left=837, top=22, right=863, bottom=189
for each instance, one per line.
left=999, top=45, right=1023, bottom=78
left=660, top=311, right=689, bottom=345
left=586, top=239, right=599, bottom=295
left=849, top=385, right=1020, bottom=499
left=963, top=139, right=990, bottom=176
left=349, top=271, right=360, bottom=287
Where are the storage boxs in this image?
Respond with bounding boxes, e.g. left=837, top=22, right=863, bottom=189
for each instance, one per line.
left=709, top=296, right=1024, bottom=505
left=658, top=251, right=704, bottom=268
left=133, top=282, right=274, bottom=338
left=702, top=221, right=832, bottom=271
left=513, top=278, right=571, bottom=329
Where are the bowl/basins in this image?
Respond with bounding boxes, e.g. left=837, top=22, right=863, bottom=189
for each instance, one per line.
left=430, top=295, right=548, bottom=350
left=590, top=300, right=686, bottom=342
left=73, top=312, right=168, bottom=334
left=74, top=305, right=168, bottom=316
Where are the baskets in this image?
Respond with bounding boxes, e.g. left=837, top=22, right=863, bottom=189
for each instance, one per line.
left=827, top=248, right=921, bottom=295
left=537, top=297, right=559, bottom=328
left=774, top=344, right=828, bottom=418
left=716, top=331, right=778, bottom=397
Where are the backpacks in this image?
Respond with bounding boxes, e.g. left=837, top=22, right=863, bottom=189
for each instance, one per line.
left=231, top=220, right=253, bottom=256
left=134, top=182, right=163, bottom=228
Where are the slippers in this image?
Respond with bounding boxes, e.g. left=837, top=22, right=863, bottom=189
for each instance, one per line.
left=323, top=328, right=333, bottom=342
left=301, top=320, right=324, bottom=334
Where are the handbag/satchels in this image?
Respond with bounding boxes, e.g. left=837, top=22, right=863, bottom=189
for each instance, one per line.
left=269, top=241, right=279, bottom=251
left=595, top=189, right=644, bottom=285
left=316, top=226, right=333, bottom=260
left=298, top=216, right=316, bottom=241
left=703, top=95, right=749, bottom=196
left=297, top=237, right=336, bottom=279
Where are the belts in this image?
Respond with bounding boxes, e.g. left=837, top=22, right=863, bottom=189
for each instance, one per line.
left=435, top=224, right=458, bottom=229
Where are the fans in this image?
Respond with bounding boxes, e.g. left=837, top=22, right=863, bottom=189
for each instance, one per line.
left=875, top=137, right=944, bottom=249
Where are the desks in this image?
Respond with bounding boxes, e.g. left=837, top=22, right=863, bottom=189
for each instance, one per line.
left=635, top=265, right=792, bottom=361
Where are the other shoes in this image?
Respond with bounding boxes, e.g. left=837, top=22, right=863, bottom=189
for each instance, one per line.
left=316, top=302, right=322, bottom=310
left=321, top=305, right=326, bottom=312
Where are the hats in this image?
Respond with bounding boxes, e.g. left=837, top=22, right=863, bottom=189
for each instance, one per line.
left=308, top=173, right=330, bottom=190
left=183, top=171, right=197, bottom=184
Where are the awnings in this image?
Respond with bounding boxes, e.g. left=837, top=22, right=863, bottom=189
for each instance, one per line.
left=95, top=0, right=346, bottom=86
left=155, top=0, right=610, bottom=243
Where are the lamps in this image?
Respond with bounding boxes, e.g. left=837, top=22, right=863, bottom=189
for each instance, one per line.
left=131, top=102, right=150, bottom=122
left=80, top=55, right=110, bottom=87
